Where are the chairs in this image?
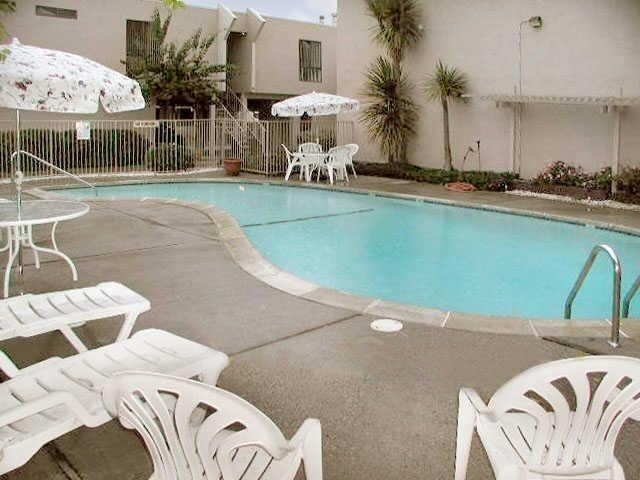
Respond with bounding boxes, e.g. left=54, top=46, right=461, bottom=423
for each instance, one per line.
left=1, top=282, right=152, bottom=378
left=106, top=370, right=329, bottom=478
left=450, top=359, right=640, bottom=480
left=0, top=326, right=229, bottom=477
left=279, top=139, right=361, bottom=186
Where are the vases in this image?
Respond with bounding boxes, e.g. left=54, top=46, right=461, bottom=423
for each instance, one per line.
left=222, top=157, right=243, bottom=177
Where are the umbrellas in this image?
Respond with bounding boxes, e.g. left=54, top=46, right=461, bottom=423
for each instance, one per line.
left=270, top=90, right=361, bottom=156
left=0, top=34, right=147, bottom=276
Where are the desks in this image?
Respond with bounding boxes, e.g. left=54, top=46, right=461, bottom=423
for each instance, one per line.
left=0, top=201, right=91, bottom=298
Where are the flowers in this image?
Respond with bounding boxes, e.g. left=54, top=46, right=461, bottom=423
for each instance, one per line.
left=525, top=161, right=640, bottom=198
